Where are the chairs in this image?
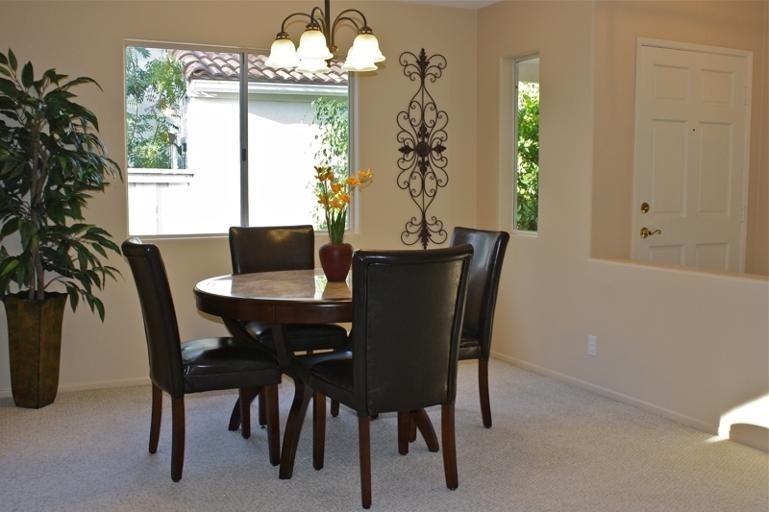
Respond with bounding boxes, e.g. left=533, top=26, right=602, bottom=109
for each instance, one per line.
left=119, top=234, right=280, bottom=482
left=278, top=243, right=474, bottom=509
left=372, top=226, right=510, bottom=443
left=227, top=225, right=347, bottom=432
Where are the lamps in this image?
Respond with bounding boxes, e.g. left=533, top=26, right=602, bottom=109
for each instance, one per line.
left=261, top=1, right=386, bottom=78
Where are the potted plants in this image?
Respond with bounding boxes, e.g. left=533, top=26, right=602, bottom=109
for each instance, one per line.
left=1, top=47, right=125, bottom=409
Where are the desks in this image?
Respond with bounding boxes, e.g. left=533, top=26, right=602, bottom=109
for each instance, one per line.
left=192, top=267, right=354, bottom=472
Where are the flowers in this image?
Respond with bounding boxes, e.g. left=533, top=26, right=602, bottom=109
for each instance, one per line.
left=313, top=165, right=373, bottom=242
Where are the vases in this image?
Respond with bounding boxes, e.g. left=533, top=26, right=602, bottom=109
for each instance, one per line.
left=318, top=243, right=353, bottom=282
